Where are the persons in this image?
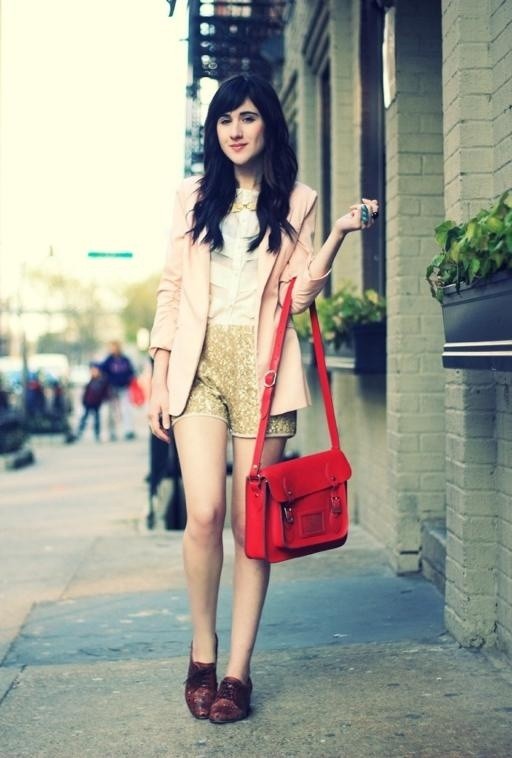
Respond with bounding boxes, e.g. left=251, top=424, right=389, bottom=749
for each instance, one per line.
left=100, top=340, right=136, bottom=441
left=148, top=75, right=380, bottom=724
left=76, top=362, right=108, bottom=442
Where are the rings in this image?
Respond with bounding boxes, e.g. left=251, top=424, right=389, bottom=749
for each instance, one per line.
left=361, top=205, right=368, bottom=224
left=371, top=212, right=379, bottom=218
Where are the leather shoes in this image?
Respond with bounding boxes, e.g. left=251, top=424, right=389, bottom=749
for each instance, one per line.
left=185, top=634, right=251, bottom=722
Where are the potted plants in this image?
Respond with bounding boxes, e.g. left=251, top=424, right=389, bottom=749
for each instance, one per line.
left=426, top=190, right=512, bottom=371
left=290, top=278, right=386, bottom=374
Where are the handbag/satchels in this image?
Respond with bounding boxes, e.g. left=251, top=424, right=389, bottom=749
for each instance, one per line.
left=246, top=448, right=353, bottom=563
